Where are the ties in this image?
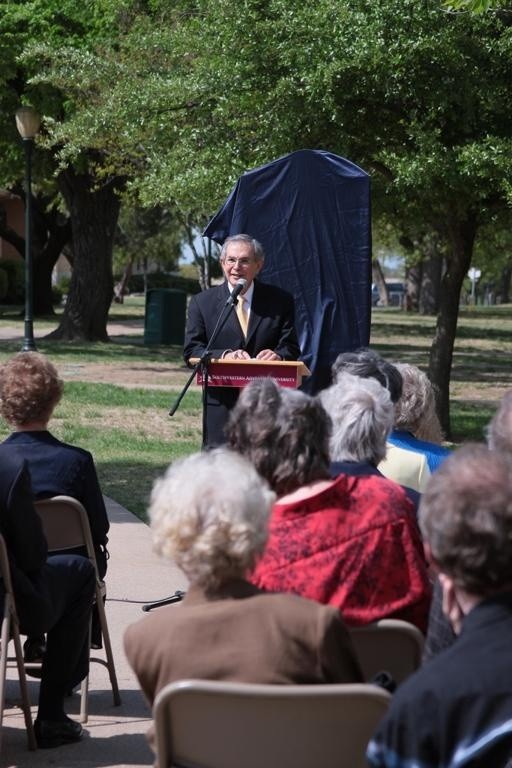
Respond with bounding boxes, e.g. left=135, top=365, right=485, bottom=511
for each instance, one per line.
left=235, top=297, right=248, bottom=338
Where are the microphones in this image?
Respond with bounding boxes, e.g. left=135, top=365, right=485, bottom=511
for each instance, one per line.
left=225, top=279, right=248, bottom=305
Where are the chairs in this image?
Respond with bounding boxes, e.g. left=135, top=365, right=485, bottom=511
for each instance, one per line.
left=1, top=495, right=125, bottom=768
left=143, top=619, right=512, bottom=767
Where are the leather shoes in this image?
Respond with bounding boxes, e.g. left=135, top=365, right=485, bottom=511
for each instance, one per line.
left=21, top=639, right=46, bottom=679
left=31, top=715, right=85, bottom=748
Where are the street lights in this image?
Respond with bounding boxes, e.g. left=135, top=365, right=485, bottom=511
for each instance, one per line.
left=15, top=107, right=41, bottom=352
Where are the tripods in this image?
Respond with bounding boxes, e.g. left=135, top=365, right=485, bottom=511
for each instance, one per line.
left=142, top=299, right=239, bottom=612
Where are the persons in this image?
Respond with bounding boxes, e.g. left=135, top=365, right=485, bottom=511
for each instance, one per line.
left=414, top=393, right=447, bottom=446
left=317, top=371, right=421, bottom=512
left=0, top=350, right=112, bottom=674
left=365, top=440, right=511, bottom=768
left=483, top=388, right=512, bottom=454
left=330, top=347, right=431, bottom=493
left=388, top=362, right=455, bottom=473
left=184, top=232, right=303, bottom=450
left=222, top=373, right=434, bottom=639
left=419, top=580, right=457, bottom=668
left=0, top=440, right=95, bottom=749
left=120, top=443, right=364, bottom=768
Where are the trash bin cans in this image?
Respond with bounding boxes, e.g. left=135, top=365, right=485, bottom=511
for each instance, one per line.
left=145, top=288, right=188, bottom=348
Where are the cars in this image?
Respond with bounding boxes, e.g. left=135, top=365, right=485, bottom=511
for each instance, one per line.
left=372, top=279, right=405, bottom=307
